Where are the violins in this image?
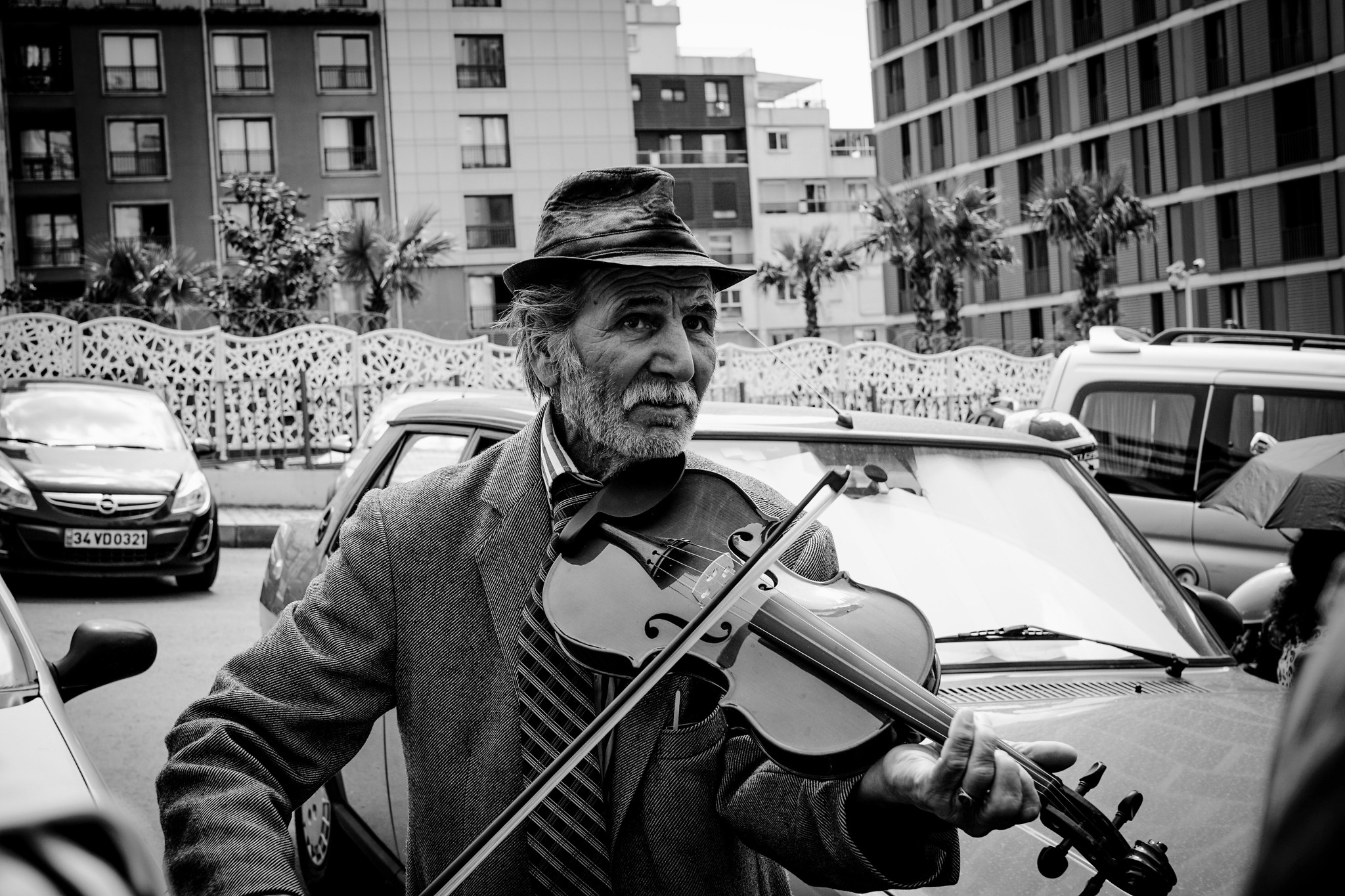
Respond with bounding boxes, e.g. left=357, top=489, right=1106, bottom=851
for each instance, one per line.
left=540, top=464, right=1179, bottom=896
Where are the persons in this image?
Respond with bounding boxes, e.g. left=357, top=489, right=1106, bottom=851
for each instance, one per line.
left=153, top=167, right=1079, bottom=896
left=1262, top=523, right=1345, bottom=687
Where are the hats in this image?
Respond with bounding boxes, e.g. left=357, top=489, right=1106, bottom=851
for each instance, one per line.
left=503, top=167, right=757, bottom=300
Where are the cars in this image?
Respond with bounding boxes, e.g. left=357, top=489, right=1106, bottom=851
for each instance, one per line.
left=0, top=375, right=221, bottom=596
left=288, top=383, right=1323, bottom=896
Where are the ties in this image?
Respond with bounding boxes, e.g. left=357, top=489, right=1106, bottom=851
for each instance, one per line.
left=515, top=476, right=613, bottom=896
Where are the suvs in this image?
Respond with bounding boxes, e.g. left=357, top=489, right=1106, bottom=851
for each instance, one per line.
left=1035, top=321, right=1345, bottom=663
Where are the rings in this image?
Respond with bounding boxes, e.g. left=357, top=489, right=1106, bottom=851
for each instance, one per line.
left=958, top=787, right=984, bottom=811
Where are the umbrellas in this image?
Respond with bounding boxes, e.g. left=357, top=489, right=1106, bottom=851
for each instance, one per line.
left=1198, top=430, right=1344, bottom=528
